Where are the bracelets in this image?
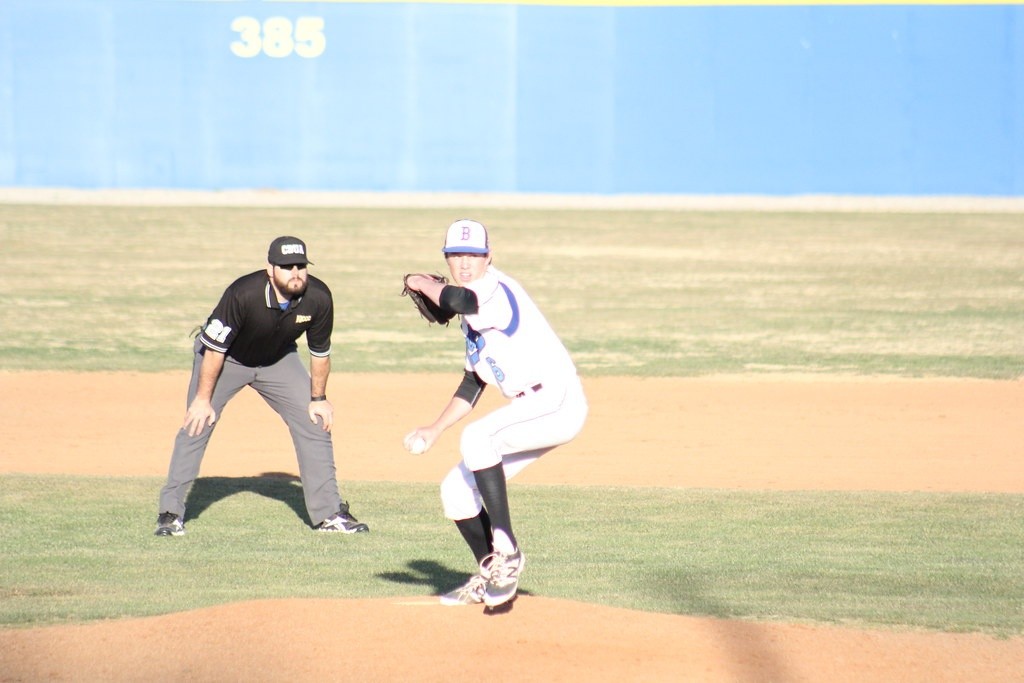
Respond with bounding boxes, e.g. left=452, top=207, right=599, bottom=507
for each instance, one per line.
left=311, top=395, right=326, bottom=401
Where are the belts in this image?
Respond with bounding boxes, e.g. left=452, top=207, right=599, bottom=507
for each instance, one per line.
left=515, top=383, right=543, bottom=398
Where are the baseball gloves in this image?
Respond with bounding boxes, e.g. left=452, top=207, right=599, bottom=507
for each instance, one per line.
left=402, top=272, right=456, bottom=325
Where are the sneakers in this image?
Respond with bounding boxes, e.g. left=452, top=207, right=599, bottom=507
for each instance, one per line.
left=316, top=501, right=370, bottom=533
left=154, top=512, right=185, bottom=536
left=479, top=547, right=524, bottom=606
left=439, top=574, right=490, bottom=605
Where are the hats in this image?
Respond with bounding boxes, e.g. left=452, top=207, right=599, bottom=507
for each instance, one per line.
left=442, top=220, right=488, bottom=253
left=268, top=236, right=314, bottom=266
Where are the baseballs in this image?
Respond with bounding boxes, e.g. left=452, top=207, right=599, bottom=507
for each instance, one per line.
left=407, top=436, right=426, bottom=454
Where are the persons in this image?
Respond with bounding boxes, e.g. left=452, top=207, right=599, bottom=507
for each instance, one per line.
left=404, top=219, right=589, bottom=605
left=152, top=235, right=369, bottom=537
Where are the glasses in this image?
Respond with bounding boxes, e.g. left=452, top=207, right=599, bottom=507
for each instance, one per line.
left=277, top=262, right=307, bottom=270
